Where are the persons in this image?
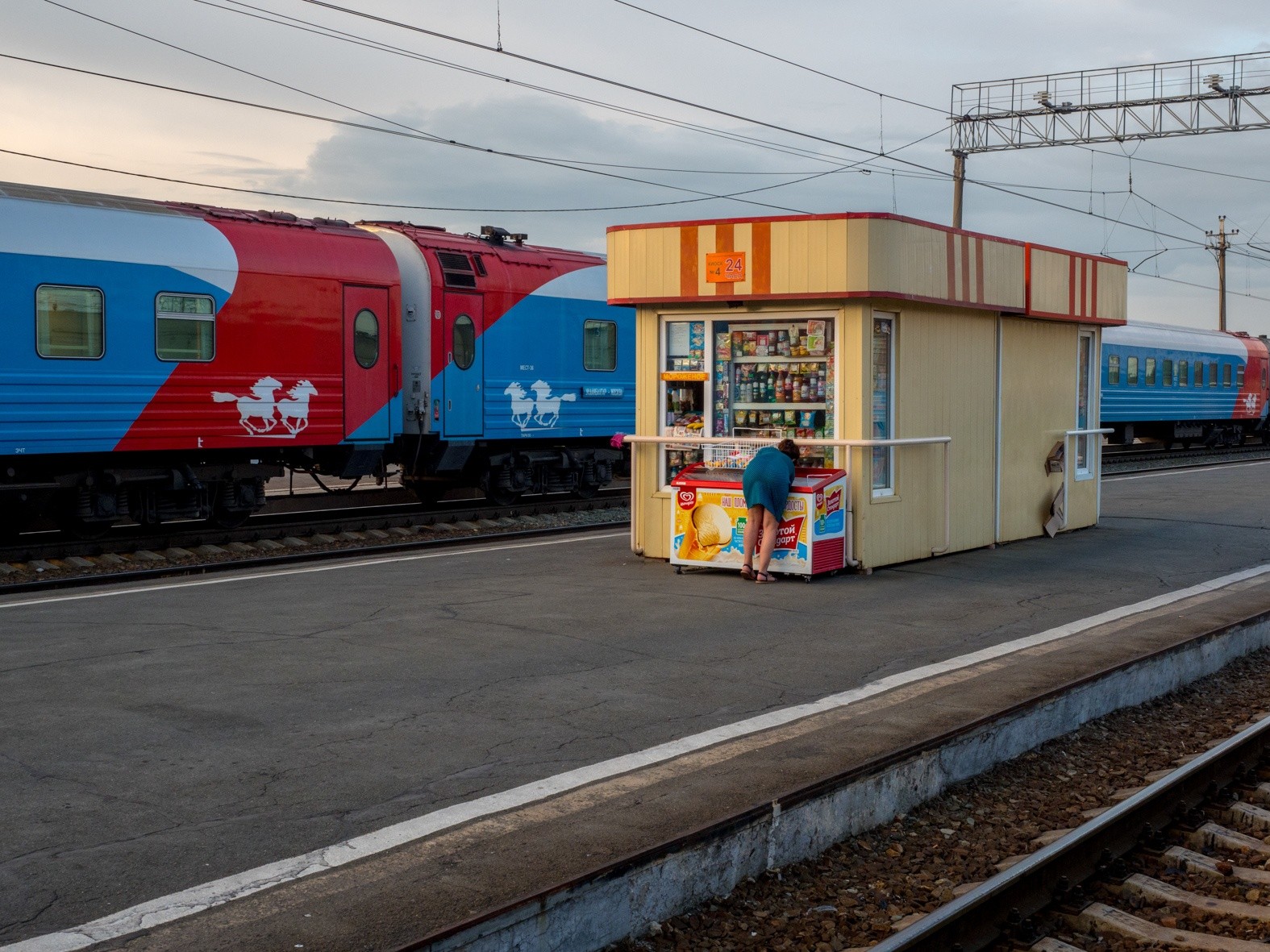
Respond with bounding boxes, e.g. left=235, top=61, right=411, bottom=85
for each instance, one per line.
left=740, top=439, right=800, bottom=584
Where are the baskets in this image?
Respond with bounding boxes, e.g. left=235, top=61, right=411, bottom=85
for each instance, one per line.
left=701, top=427, right=783, bottom=470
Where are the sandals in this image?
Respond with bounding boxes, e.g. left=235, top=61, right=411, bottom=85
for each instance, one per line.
left=740, top=564, right=757, bottom=580
left=755, top=572, right=778, bottom=583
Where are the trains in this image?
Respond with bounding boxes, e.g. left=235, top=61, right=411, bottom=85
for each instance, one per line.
left=1, top=178, right=1270, bottom=523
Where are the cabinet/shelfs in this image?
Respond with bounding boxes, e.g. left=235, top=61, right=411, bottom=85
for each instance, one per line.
left=728, top=321, right=835, bottom=470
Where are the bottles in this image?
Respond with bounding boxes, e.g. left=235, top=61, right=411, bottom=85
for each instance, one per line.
left=733, top=366, right=826, bottom=404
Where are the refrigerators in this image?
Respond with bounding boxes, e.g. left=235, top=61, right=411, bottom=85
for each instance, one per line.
left=670, top=464, right=847, bottom=583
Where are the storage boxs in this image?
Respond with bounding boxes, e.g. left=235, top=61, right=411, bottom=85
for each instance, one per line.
left=701, top=428, right=784, bottom=471
